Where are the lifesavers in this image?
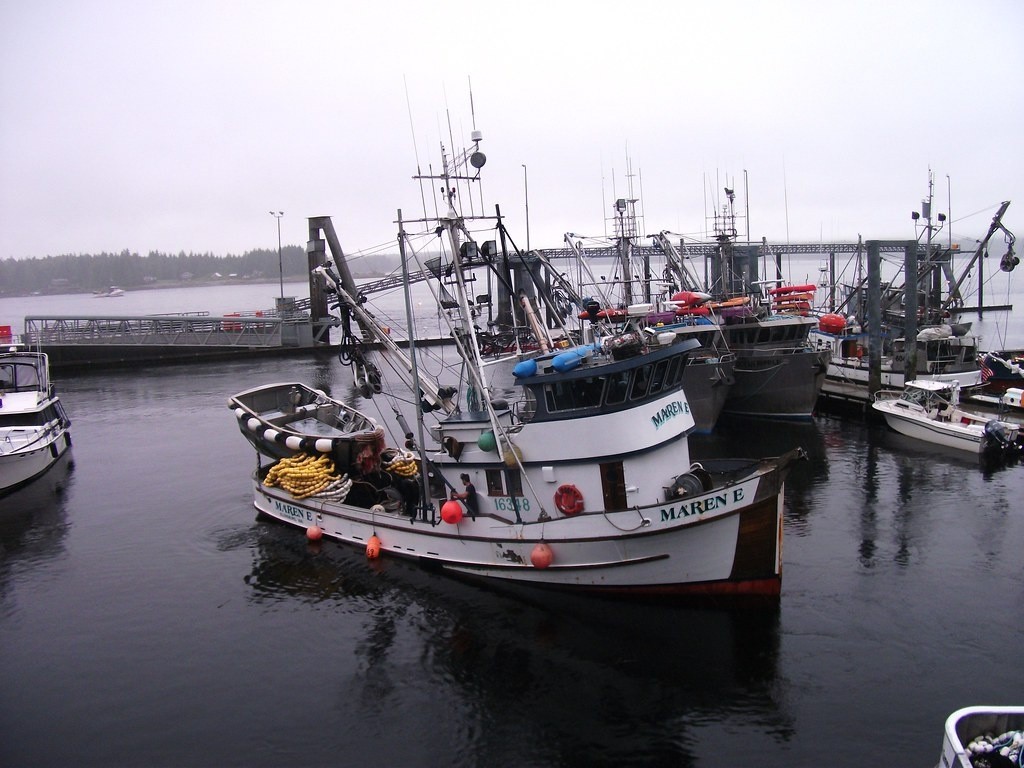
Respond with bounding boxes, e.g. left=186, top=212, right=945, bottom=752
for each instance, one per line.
left=555, top=485, right=583, bottom=516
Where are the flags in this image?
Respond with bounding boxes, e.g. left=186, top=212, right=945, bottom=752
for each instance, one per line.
left=980, top=355, right=994, bottom=380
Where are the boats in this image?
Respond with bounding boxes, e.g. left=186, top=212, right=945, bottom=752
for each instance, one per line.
left=106, top=285, right=125, bottom=297
left=0, top=344, right=72, bottom=499
left=227, top=379, right=384, bottom=469
left=254, top=75, right=807, bottom=603
left=450, top=156, right=1024, bottom=458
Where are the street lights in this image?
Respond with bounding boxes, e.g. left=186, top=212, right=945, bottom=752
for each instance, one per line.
left=268, top=209, right=286, bottom=297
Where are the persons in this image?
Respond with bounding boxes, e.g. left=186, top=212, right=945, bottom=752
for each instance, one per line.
left=450, top=473, right=479, bottom=516
